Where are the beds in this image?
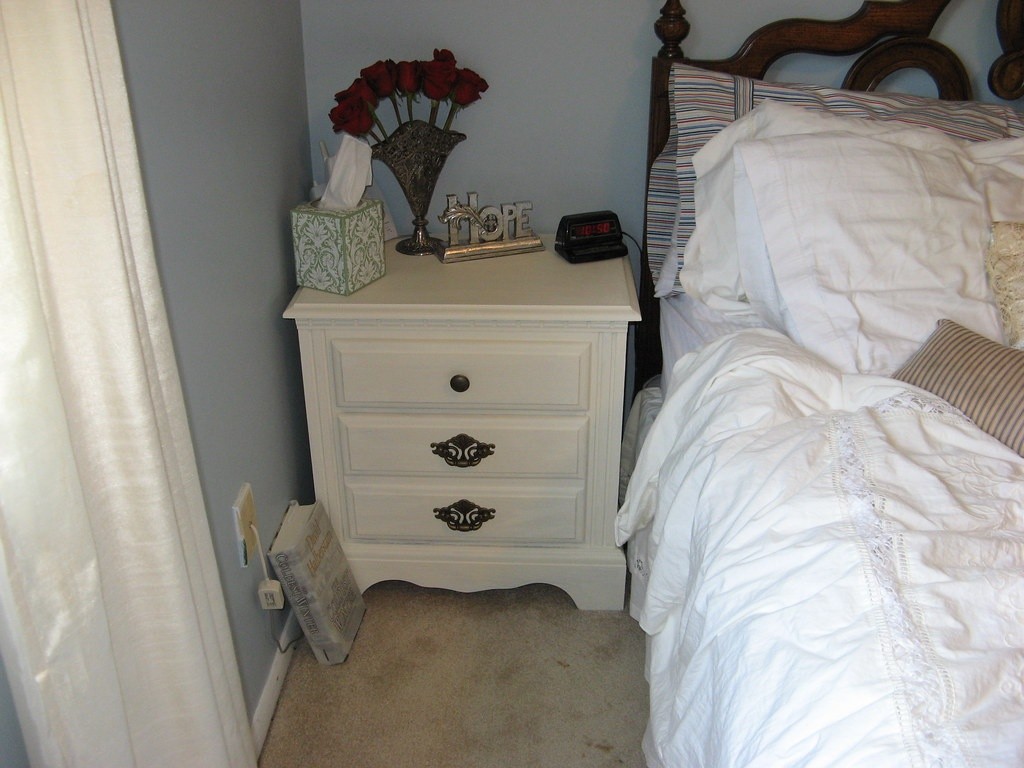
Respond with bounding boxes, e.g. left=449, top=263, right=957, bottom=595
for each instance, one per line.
left=612, top=0, right=1024, bottom=768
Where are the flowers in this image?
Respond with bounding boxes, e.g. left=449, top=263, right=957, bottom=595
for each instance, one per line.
left=328, top=48, right=488, bottom=143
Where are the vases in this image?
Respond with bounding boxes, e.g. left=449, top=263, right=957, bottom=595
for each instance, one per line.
left=370, top=120, right=466, bottom=257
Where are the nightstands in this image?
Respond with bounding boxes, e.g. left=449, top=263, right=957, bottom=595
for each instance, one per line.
left=282, top=233, right=642, bottom=611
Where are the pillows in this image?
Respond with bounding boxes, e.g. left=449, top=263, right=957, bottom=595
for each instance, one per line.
left=647, top=62, right=1024, bottom=299
left=892, top=317, right=1024, bottom=458
left=682, top=100, right=1024, bottom=310
left=732, top=138, right=1024, bottom=377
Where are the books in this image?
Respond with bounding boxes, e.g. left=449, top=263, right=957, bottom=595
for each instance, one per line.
left=268, top=501, right=366, bottom=666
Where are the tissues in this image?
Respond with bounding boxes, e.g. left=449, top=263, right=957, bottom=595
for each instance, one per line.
left=290, top=135, right=386, bottom=297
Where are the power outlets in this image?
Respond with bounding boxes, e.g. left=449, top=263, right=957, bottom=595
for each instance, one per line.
left=233, top=483, right=257, bottom=567
